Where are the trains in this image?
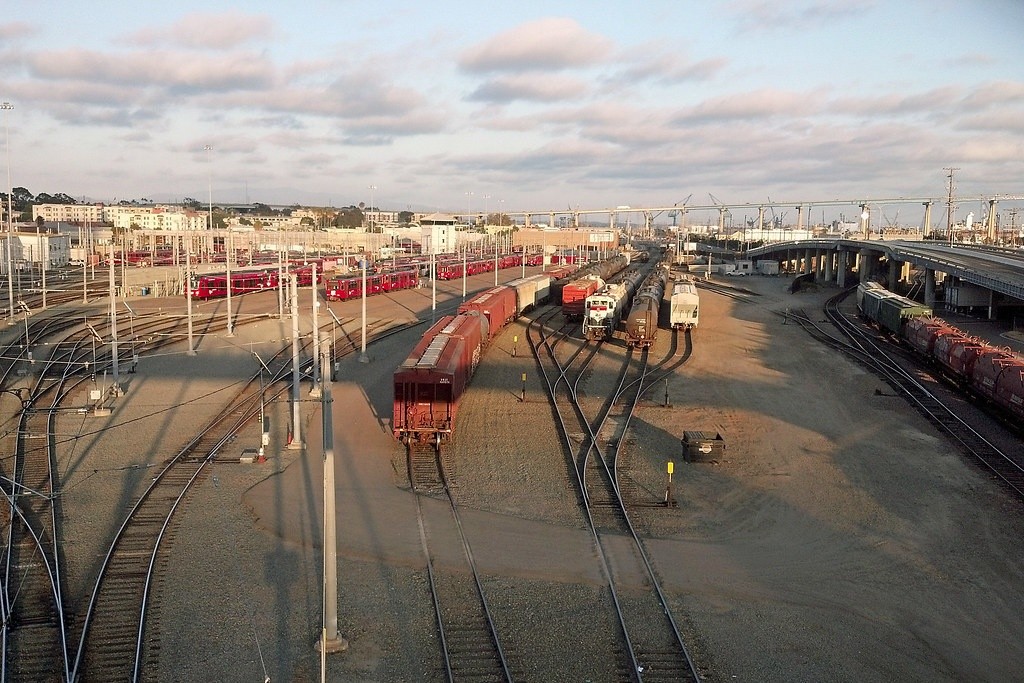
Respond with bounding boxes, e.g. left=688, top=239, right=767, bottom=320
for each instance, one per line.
left=99, top=241, right=577, bottom=303
left=550, top=248, right=669, bottom=349
left=391, top=264, right=579, bottom=449
left=671, top=273, right=700, bottom=330
left=856, top=271, right=1023, bottom=422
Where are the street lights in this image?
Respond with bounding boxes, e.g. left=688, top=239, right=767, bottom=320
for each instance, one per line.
left=367, top=185, right=378, bottom=262
left=0, top=99, right=19, bottom=326
left=204, top=144, right=214, bottom=263
left=465, top=191, right=474, bottom=252
left=497, top=198, right=505, bottom=254
left=479, top=194, right=491, bottom=259
